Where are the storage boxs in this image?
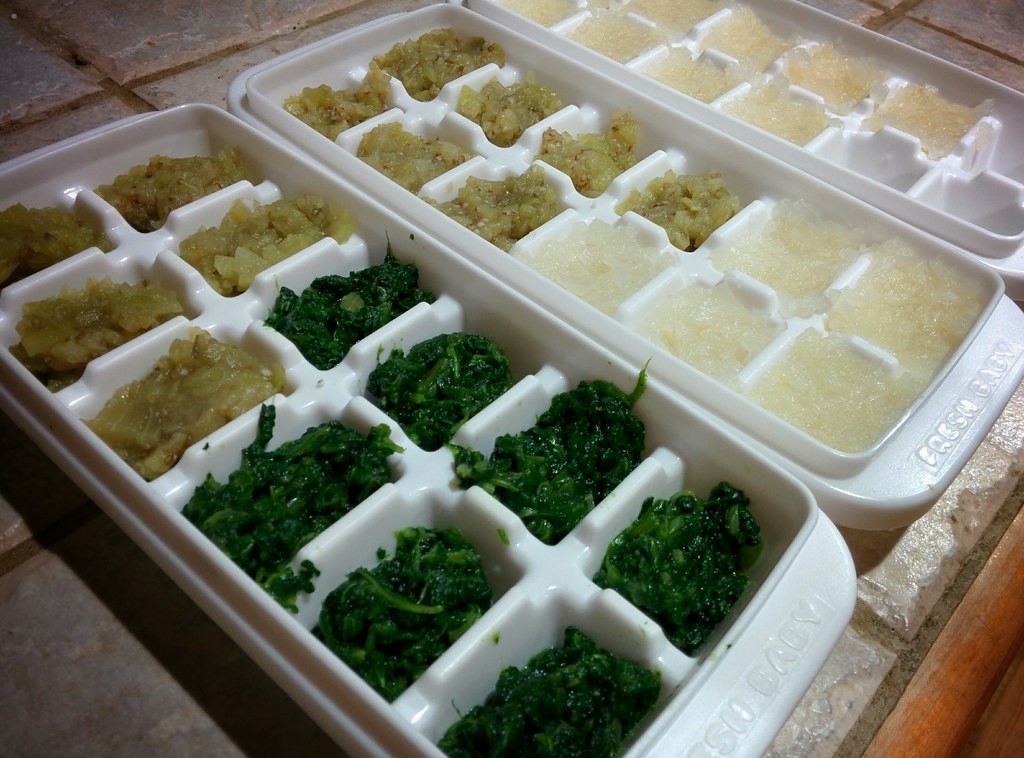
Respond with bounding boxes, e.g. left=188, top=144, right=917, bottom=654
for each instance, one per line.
left=0, top=0, right=1024, bottom=758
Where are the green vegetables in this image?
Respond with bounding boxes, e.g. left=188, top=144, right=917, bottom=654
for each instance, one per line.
left=186, top=242, right=764, bottom=758
